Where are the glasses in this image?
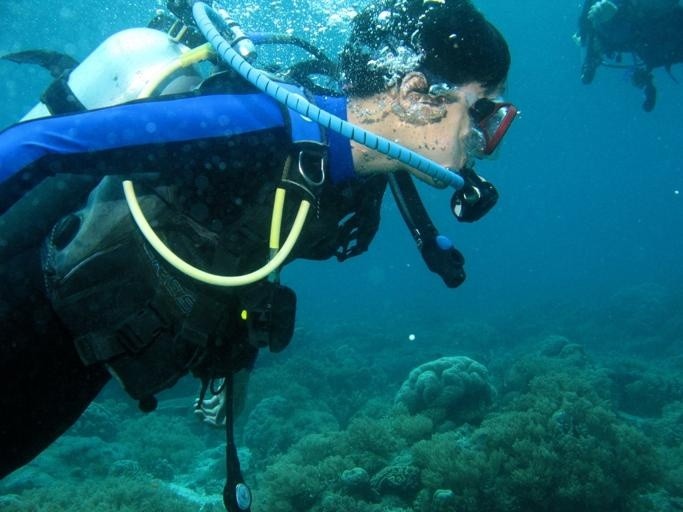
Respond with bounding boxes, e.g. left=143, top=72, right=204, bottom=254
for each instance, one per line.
left=419, top=84, right=518, bottom=159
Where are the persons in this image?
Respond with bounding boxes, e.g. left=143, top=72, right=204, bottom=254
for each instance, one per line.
left=0, top=1, right=519, bottom=481
left=568, top=0, right=683, bottom=113
